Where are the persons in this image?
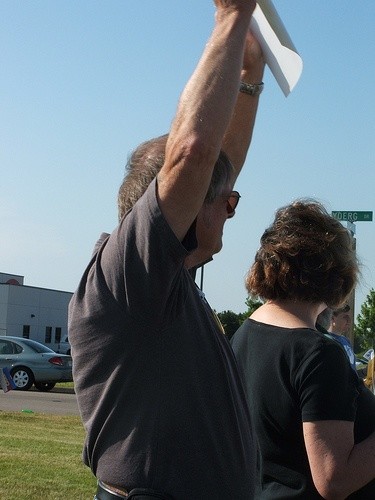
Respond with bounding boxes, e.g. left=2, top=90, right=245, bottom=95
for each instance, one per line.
left=229, top=197, right=375, bottom=500
left=69, top=0, right=267, bottom=500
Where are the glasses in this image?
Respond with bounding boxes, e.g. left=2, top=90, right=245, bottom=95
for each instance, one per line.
left=221, top=191, right=241, bottom=213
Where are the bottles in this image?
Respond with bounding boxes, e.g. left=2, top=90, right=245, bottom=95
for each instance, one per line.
left=21, top=410, right=32, bottom=413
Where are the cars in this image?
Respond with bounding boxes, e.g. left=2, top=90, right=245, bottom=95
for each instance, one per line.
left=0, top=336, right=74, bottom=392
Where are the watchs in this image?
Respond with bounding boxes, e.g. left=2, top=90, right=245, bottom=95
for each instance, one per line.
left=239, top=81, right=265, bottom=96
